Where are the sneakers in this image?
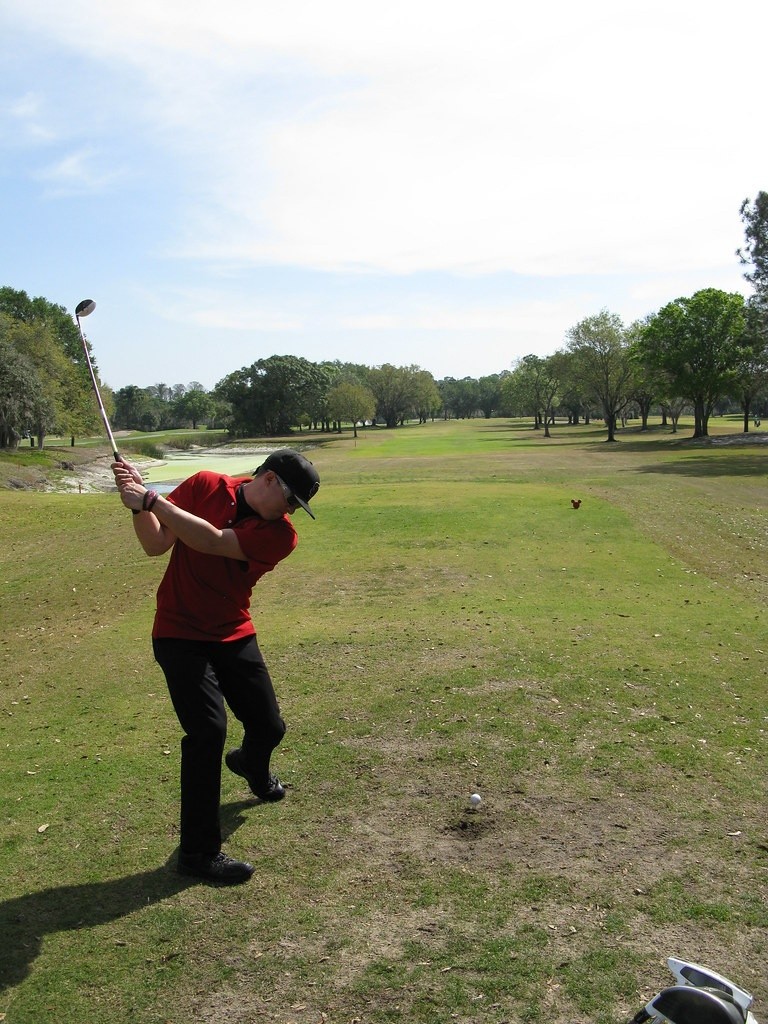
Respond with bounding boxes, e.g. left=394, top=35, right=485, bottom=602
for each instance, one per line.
left=225, top=746, right=286, bottom=800
left=177, top=843, right=256, bottom=881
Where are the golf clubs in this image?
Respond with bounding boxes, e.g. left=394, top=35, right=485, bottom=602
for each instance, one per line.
left=74, top=297, right=141, bottom=515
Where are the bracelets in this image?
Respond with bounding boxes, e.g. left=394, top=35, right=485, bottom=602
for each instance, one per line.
left=143, top=490, right=159, bottom=511
left=131, top=509, right=141, bottom=514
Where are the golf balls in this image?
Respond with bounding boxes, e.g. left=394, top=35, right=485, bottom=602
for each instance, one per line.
left=471, top=793, right=481, bottom=804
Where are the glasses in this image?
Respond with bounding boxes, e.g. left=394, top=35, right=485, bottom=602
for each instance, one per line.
left=267, top=468, right=301, bottom=509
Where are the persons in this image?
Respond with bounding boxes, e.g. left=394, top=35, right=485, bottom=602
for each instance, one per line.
left=111, top=448, right=320, bottom=886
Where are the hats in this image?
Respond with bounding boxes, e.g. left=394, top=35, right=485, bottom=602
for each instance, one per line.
left=264, top=449, right=320, bottom=520
